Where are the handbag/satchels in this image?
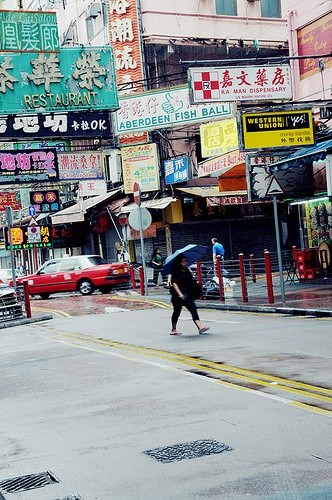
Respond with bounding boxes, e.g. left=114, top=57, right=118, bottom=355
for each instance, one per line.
left=193, top=279, right=202, bottom=300
left=149, top=256, right=158, bottom=269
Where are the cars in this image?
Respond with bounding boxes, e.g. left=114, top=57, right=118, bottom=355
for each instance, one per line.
left=10, top=254, right=129, bottom=296
left=0, top=268, right=24, bottom=285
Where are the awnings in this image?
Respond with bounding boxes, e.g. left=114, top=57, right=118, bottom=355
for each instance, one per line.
left=267, top=139, right=332, bottom=171
left=49, top=190, right=121, bottom=224
left=176, top=185, right=285, bottom=206
left=115, top=196, right=178, bottom=217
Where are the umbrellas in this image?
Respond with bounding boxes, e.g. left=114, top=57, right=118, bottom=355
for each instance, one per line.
left=163, top=244, right=207, bottom=267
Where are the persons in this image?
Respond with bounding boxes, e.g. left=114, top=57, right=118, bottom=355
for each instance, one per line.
left=152, top=247, right=167, bottom=287
left=16, top=262, right=26, bottom=274
left=212, top=238, right=225, bottom=278
left=169, top=255, right=209, bottom=335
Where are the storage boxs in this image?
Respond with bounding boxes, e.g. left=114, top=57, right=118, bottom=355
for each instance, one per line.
left=294, top=247, right=322, bottom=278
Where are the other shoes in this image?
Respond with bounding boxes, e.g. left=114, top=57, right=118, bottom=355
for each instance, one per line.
left=170, top=331, right=181, bottom=334
left=199, top=327, right=209, bottom=334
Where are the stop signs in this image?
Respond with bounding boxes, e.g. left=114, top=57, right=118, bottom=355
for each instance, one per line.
left=133, top=182, right=141, bottom=206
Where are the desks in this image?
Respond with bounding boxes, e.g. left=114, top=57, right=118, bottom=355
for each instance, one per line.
left=282, top=259, right=300, bottom=285
